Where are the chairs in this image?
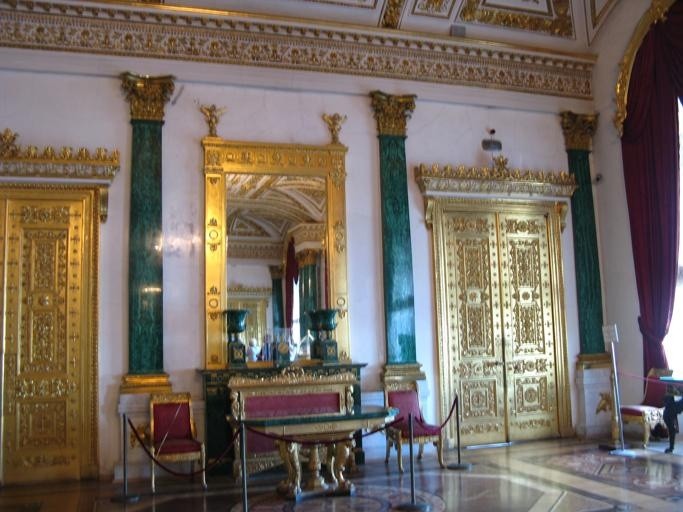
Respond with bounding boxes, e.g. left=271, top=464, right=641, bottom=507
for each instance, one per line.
left=148, top=390, right=210, bottom=493
left=381, top=380, right=447, bottom=474
left=619, top=366, right=674, bottom=449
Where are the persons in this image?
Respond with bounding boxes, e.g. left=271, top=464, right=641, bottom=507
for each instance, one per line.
left=255, top=334, right=273, bottom=360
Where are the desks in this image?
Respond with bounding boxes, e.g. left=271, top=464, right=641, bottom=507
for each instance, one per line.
left=236, top=403, right=400, bottom=503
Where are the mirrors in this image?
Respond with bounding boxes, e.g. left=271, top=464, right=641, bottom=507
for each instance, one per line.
left=196, top=101, right=353, bottom=372
